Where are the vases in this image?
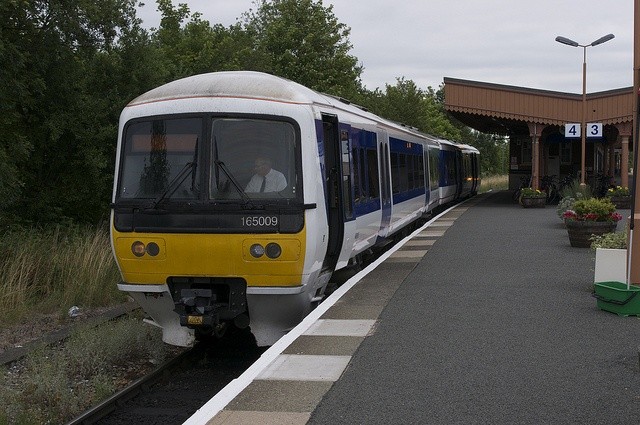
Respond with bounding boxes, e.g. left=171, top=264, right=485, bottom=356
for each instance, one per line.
left=611, top=196, right=630, bottom=208
left=521, top=196, right=546, bottom=208
left=566, top=221, right=617, bottom=248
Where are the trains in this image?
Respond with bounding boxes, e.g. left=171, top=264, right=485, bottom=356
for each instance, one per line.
left=110, top=70, right=481, bottom=349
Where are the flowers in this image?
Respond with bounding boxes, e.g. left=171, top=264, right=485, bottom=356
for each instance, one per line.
left=556, top=192, right=623, bottom=222
left=522, top=188, right=547, bottom=197
left=608, top=186, right=632, bottom=196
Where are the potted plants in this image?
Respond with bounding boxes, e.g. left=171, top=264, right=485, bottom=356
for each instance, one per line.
left=587, top=230, right=629, bottom=283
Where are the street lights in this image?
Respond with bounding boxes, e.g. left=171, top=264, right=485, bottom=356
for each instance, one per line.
left=556, top=34, right=615, bottom=190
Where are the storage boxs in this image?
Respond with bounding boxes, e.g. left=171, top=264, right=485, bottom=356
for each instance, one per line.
left=590, top=280, right=640, bottom=318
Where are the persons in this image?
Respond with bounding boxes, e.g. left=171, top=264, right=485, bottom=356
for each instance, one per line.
left=243, top=155, right=288, bottom=193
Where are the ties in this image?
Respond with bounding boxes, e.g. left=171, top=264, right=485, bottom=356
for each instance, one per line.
left=260, top=176, right=266, bottom=192
